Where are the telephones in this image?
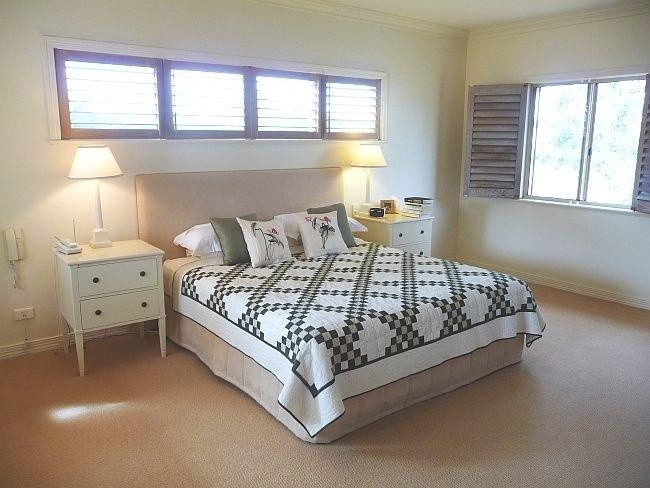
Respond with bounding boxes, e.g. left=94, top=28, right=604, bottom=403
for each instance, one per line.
left=4, top=227, right=24, bottom=263
left=55, top=235, right=82, bottom=255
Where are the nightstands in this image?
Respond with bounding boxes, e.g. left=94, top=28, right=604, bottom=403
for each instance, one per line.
left=354, top=215, right=434, bottom=257
left=53, top=238, right=166, bottom=376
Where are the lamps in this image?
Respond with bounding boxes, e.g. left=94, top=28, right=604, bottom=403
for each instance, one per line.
left=67, top=144, right=124, bottom=248
left=351, top=143, right=387, bottom=217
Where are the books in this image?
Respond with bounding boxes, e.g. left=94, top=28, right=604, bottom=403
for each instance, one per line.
left=402, top=197, right=434, bottom=218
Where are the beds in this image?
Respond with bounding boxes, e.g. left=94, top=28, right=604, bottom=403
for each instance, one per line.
left=134, top=167, right=546, bottom=444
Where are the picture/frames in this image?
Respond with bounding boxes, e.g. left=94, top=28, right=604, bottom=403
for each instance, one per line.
left=380, top=200, right=395, bottom=214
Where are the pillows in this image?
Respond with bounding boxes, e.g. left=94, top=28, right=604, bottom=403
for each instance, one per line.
left=173, top=222, right=222, bottom=259
left=210, top=213, right=258, bottom=265
left=294, top=210, right=349, bottom=260
left=274, top=212, right=368, bottom=245
left=236, top=216, right=292, bottom=268
left=307, top=203, right=357, bottom=247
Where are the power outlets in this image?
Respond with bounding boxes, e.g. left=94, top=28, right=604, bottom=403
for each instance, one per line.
left=13, top=307, right=34, bottom=321
